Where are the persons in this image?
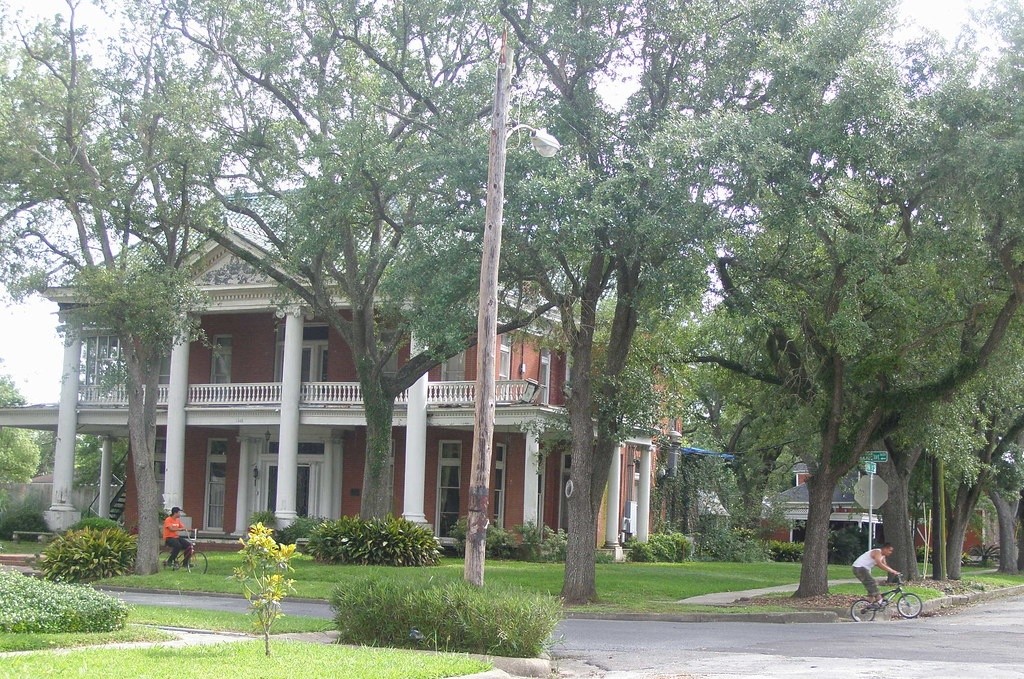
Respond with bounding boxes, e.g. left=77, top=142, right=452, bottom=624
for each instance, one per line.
left=163, top=507, right=198, bottom=567
left=852, top=542, right=903, bottom=607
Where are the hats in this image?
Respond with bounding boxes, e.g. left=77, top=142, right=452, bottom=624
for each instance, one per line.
left=171, top=506, right=184, bottom=512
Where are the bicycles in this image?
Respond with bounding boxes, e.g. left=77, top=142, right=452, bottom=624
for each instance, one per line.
left=850, top=574, right=923, bottom=623
left=159, top=528, right=209, bottom=575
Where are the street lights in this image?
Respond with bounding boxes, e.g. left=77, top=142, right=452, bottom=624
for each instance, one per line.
left=461, top=124, right=563, bottom=584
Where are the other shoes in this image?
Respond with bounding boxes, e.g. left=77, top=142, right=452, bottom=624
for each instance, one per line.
left=182, top=563, right=195, bottom=567
left=167, top=559, right=172, bottom=567
left=878, top=599, right=889, bottom=608
